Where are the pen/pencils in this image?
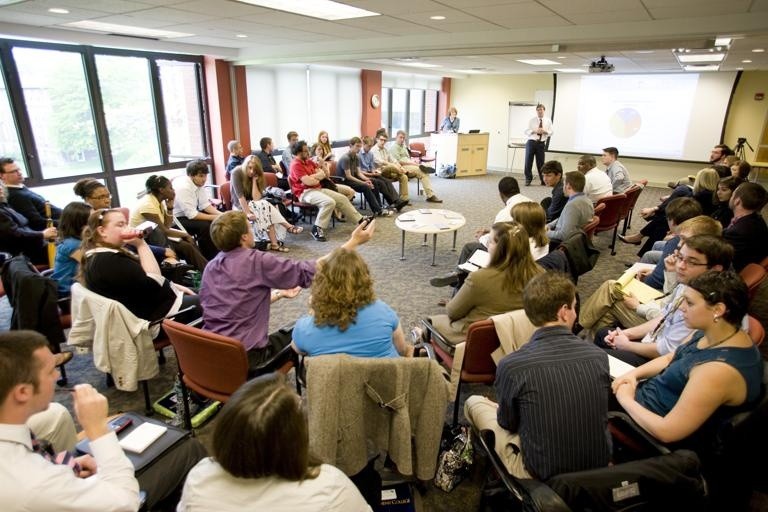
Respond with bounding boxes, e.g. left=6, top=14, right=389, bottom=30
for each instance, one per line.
left=55, top=388, right=78, bottom=391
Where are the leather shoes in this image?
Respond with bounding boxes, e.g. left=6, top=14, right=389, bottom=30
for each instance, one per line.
left=429, top=272, right=459, bottom=288
left=617, top=233, right=642, bottom=245
left=427, top=194, right=444, bottom=204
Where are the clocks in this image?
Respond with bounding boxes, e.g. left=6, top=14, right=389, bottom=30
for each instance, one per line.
left=372, top=95, right=379, bottom=108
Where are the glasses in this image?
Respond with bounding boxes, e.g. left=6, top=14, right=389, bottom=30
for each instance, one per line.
left=89, top=193, right=114, bottom=200
left=676, top=255, right=710, bottom=267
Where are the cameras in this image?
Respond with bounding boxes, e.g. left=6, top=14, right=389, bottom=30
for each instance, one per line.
left=739, top=138, right=746, bottom=143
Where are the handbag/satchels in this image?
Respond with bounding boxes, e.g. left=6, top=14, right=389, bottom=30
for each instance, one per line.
left=433, top=425, right=476, bottom=493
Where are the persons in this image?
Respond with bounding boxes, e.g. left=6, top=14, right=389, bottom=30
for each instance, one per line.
left=408, top=220, right=545, bottom=363
left=230, top=155, right=303, bottom=253
left=171, top=159, right=226, bottom=261
left=226, top=131, right=443, bottom=222
left=1, top=331, right=143, bottom=512
left=77, top=209, right=202, bottom=341
left=1, top=182, right=61, bottom=265
left=177, top=374, right=373, bottom=512
left=608, top=271, right=767, bottom=484
left=428, top=146, right=768, bottom=374
left=129, top=175, right=210, bottom=273
left=461, top=271, right=610, bottom=481
left=74, top=177, right=202, bottom=288
left=200, top=210, right=375, bottom=386
left=440, top=108, right=459, bottom=133
left=288, top=140, right=375, bottom=241
left=1, top=156, right=64, bottom=241
left=524, top=104, right=553, bottom=186
left=49, top=203, right=100, bottom=312
left=292, top=249, right=411, bottom=360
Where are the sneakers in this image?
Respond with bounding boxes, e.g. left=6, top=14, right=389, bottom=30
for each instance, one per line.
left=359, top=214, right=376, bottom=228
left=309, top=225, right=327, bottom=242
left=373, top=200, right=409, bottom=217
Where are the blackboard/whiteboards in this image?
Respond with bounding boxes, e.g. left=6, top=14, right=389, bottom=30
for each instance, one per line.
left=509, top=103, right=539, bottom=148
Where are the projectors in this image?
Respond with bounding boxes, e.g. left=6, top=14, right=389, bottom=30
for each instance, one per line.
left=589, top=63, right=614, bottom=72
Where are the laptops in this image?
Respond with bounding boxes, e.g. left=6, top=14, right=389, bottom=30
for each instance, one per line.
left=469, top=130, right=480, bottom=133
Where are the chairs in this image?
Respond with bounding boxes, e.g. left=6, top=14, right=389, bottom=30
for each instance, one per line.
left=0, top=143, right=768, bottom=512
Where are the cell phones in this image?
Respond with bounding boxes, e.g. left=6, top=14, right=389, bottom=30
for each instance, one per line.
left=109, top=415, right=132, bottom=433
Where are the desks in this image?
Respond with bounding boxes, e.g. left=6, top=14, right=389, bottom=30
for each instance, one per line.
left=395, top=209, right=465, bottom=266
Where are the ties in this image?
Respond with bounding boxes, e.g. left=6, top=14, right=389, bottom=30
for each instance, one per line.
left=537, top=119, right=543, bottom=141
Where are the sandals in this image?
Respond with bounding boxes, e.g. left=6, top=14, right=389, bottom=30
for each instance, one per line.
left=287, top=224, right=303, bottom=235
left=271, top=241, right=290, bottom=253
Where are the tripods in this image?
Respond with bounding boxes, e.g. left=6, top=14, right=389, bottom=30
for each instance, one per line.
left=733, top=144, right=750, bottom=183
